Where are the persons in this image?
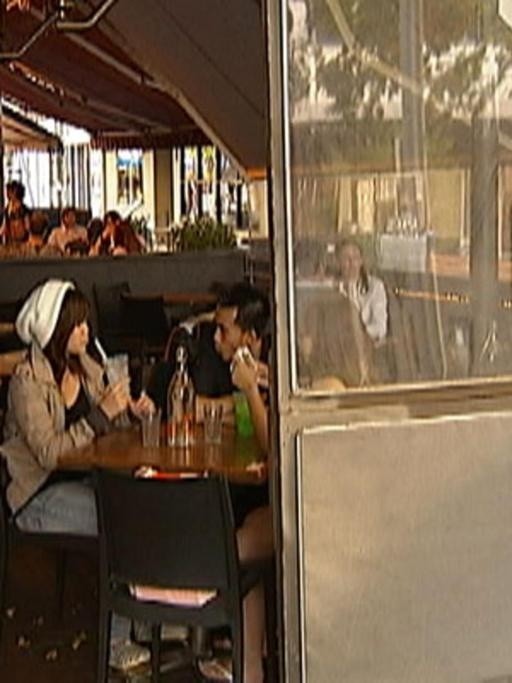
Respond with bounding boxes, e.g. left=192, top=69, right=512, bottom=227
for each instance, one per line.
left=329, top=237, right=389, bottom=343
left=1, top=276, right=189, bottom=670
left=194, top=283, right=392, bottom=683
left=291, top=236, right=334, bottom=287
left=170, top=280, right=270, bottom=529
left=0, top=177, right=148, bottom=263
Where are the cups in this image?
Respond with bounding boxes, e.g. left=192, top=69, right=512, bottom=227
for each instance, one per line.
left=102, top=355, right=254, bottom=449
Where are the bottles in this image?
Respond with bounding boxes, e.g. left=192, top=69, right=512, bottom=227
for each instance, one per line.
left=166, top=347, right=195, bottom=449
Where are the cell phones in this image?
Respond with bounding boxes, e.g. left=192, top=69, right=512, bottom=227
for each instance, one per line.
left=239, top=346, right=261, bottom=384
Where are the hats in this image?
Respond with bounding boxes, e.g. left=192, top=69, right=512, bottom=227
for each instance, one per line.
left=15, top=278, right=76, bottom=349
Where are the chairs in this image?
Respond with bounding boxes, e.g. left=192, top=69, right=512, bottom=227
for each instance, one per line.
left=1, top=221, right=499, bottom=683
